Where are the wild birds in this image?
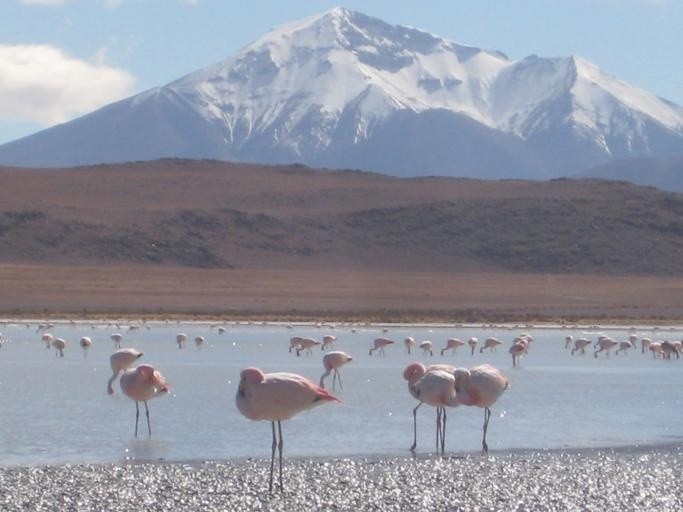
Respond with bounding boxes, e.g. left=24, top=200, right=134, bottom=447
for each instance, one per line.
left=195, top=336, right=205, bottom=349
left=399, top=361, right=509, bottom=459
left=233, top=367, right=343, bottom=492
left=79, top=336, right=92, bottom=360
left=561, top=334, right=683, bottom=361
left=104, top=349, right=144, bottom=395
left=119, top=364, right=172, bottom=437
left=217, top=326, right=228, bottom=336
left=35, top=321, right=66, bottom=357
left=88, top=318, right=151, bottom=349
left=175, top=333, right=189, bottom=348
left=317, top=350, right=353, bottom=390
left=286, top=328, right=534, bottom=369
